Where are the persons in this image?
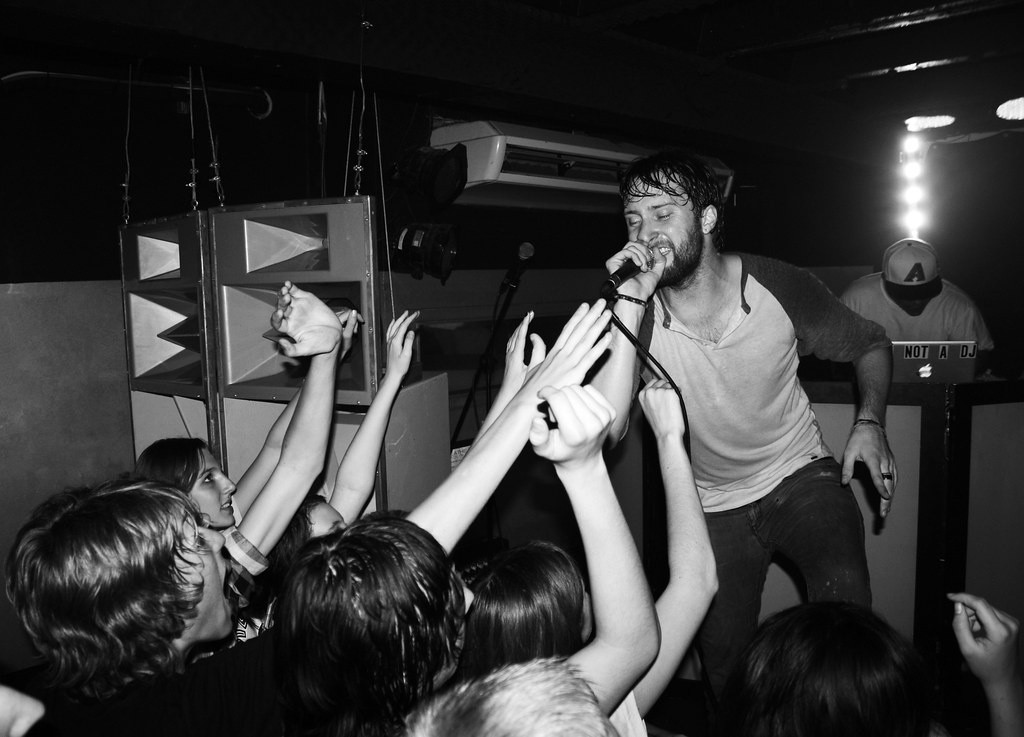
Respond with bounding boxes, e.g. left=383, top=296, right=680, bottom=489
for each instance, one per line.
left=0, top=282, right=1024, bottom=737
left=836, top=237, right=994, bottom=353
left=588, top=153, right=899, bottom=681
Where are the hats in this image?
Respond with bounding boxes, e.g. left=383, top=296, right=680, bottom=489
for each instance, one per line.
left=882, top=240, right=942, bottom=300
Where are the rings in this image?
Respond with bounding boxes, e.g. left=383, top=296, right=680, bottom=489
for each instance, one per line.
left=882, top=473, right=892, bottom=480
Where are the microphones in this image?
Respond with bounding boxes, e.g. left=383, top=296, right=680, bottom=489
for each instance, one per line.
left=500, top=243, right=534, bottom=296
left=601, top=248, right=654, bottom=294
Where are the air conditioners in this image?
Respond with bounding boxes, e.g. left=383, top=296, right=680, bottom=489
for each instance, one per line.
left=431, top=120, right=734, bottom=212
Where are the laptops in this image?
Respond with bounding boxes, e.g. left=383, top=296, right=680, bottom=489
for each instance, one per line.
left=892, top=341, right=977, bottom=381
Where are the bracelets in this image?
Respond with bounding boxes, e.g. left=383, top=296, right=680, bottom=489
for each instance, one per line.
left=853, top=419, right=887, bottom=429
left=618, top=293, right=648, bottom=308
